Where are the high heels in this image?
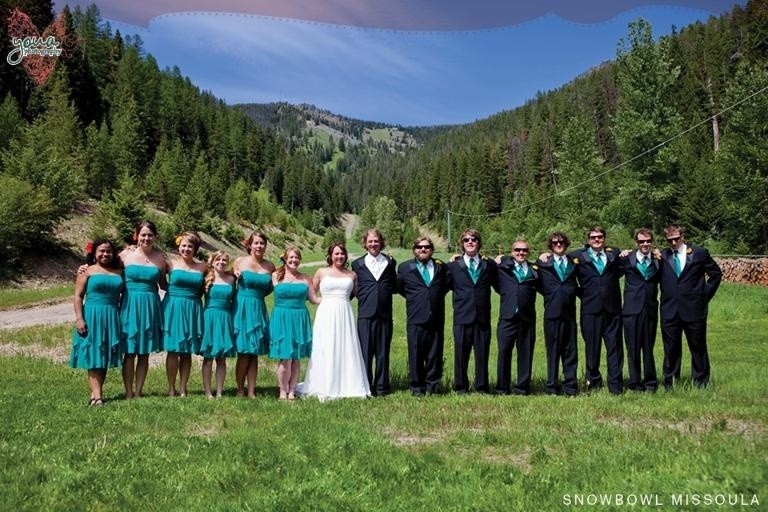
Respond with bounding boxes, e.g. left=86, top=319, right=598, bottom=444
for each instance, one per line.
left=88, top=397, right=104, bottom=408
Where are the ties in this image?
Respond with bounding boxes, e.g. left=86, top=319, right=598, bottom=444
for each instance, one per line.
left=470, top=258, right=476, bottom=283
left=597, top=253, right=604, bottom=275
left=642, top=256, right=648, bottom=280
left=420, top=262, right=431, bottom=287
left=673, top=250, right=681, bottom=276
left=517, top=264, right=524, bottom=283
left=667, top=237, right=681, bottom=244
left=558, top=257, right=566, bottom=281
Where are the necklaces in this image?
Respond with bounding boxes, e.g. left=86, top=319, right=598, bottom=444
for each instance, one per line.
left=143, top=253, right=154, bottom=264
left=177, top=258, right=194, bottom=271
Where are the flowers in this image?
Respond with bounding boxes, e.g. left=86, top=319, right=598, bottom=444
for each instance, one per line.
left=532, top=264, right=540, bottom=271
left=85, top=241, right=94, bottom=253
left=573, top=258, right=580, bottom=265
left=176, top=235, right=183, bottom=246
left=606, top=247, right=613, bottom=253
left=482, top=255, right=489, bottom=259
left=686, top=248, right=693, bottom=254
left=435, top=259, right=442, bottom=265
left=132, top=232, right=138, bottom=241
left=656, top=255, right=662, bottom=261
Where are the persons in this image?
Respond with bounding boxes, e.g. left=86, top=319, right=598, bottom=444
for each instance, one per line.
left=67, top=237, right=124, bottom=406
left=225, top=229, right=278, bottom=399
left=75, top=219, right=169, bottom=398
left=397, top=235, right=452, bottom=395
left=538, top=223, right=625, bottom=396
left=195, top=250, right=237, bottom=398
left=617, top=228, right=663, bottom=393
left=264, top=245, right=323, bottom=402
left=446, top=227, right=500, bottom=395
left=125, top=229, right=243, bottom=398
left=493, top=230, right=582, bottom=397
left=449, top=237, right=545, bottom=395
left=304, top=240, right=359, bottom=401
left=619, top=223, right=722, bottom=392
left=349, top=226, right=397, bottom=397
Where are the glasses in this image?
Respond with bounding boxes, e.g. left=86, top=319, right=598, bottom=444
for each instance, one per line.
left=513, top=248, right=529, bottom=252
left=590, top=236, right=604, bottom=240
left=552, top=241, right=566, bottom=245
left=462, top=238, right=478, bottom=242
left=638, top=240, right=652, bottom=243
left=415, top=245, right=432, bottom=249
